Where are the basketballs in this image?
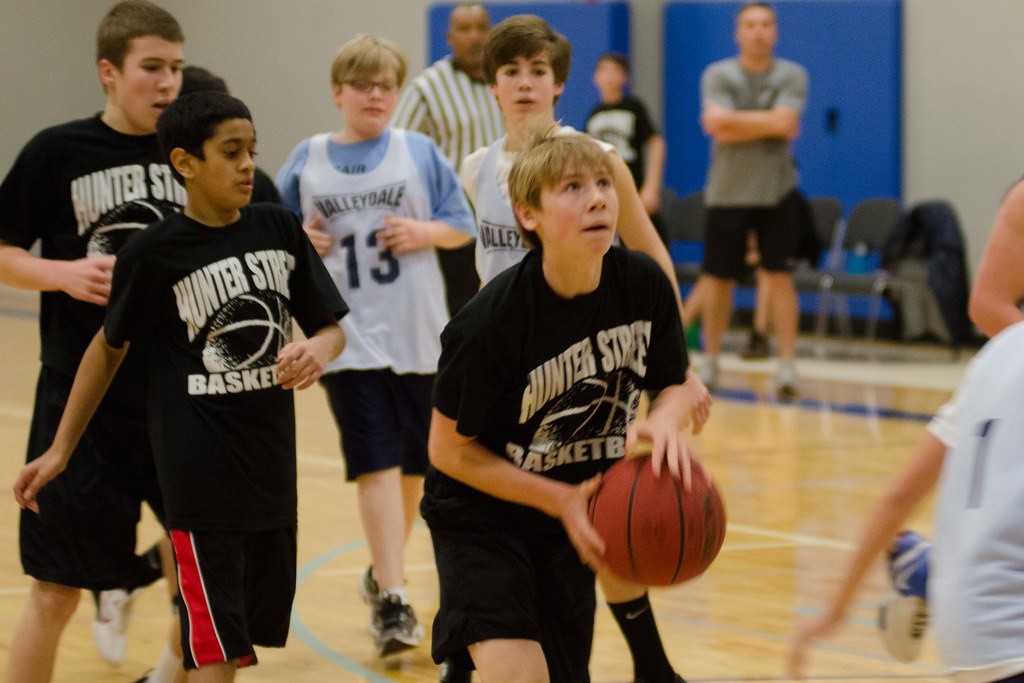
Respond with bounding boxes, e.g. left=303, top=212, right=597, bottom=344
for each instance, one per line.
left=588, top=451, right=727, bottom=585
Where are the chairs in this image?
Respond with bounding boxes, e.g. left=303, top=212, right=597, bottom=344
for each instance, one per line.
left=654, top=189, right=970, bottom=361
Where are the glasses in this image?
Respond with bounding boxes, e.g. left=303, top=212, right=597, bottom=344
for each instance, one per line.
left=340, top=77, right=399, bottom=94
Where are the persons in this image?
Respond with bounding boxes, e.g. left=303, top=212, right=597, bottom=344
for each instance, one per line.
left=1, top=1, right=1023, bottom=683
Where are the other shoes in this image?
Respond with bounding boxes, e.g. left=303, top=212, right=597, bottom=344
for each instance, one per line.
left=699, top=362, right=719, bottom=391
left=742, top=330, right=768, bottom=360
left=774, top=375, right=795, bottom=405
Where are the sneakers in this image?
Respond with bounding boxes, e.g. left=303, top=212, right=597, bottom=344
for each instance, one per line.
left=877, top=530, right=930, bottom=667
left=93, top=588, right=136, bottom=667
left=369, top=594, right=425, bottom=658
left=357, top=567, right=380, bottom=602
